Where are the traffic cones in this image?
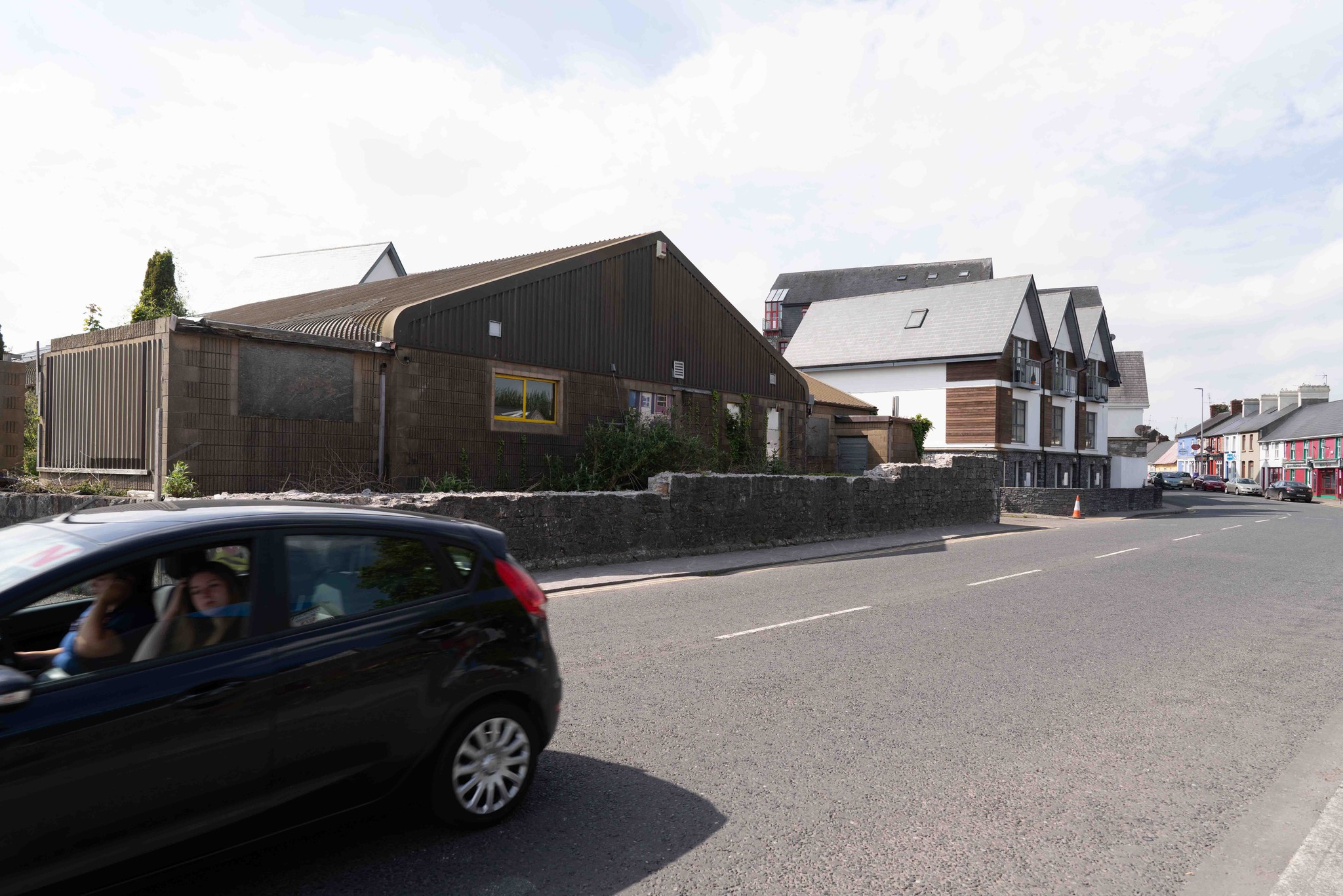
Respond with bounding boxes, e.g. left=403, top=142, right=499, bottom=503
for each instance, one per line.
left=1068, top=494, right=1085, bottom=519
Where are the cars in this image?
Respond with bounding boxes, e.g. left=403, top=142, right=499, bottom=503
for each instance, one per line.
left=1193, top=474, right=1226, bottom=492
left=1224, top=477, right=1263, bottom=497
left=0, top=498, right=563, bottom=896
left=1151, top=471, right=1184, bottom=490
left=1264, top=480, right=1313, bottom=503
left=1174, top=471, right=1193, bottom=487
left=1147, top=471, right=1158, bottom=483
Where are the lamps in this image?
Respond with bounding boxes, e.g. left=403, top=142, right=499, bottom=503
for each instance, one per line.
left=1073, top=458, right=1077, bottom=462
left=1037, top=454, right=1041, bottom=461
left=1104, top=459, right=1107, bottom=464
left=775, top=403, right=781, bottom=412
left=1180, top=442, right=1181, bottom=444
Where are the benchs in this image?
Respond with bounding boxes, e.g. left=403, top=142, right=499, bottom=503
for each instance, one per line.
left=314, top=567, right=404, bottom=614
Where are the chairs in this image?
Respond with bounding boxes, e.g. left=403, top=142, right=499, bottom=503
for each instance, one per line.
left=1242, top=480, right=1245, bottom=483
left=1182, top=474, right=1185, bottom=476
left=150, top=546, right=207, bottom=629
left=240, top=548, right=313, bottom=638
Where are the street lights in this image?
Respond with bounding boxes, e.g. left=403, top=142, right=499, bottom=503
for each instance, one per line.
left=1193, top=388, right=1204, bottom=474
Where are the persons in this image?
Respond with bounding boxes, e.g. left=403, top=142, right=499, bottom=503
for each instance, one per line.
left=15, top=561, right=158, bottom=684
left=131, top=563, right=249, bottom=664
left=1192, top=469, right=1199, bottom=479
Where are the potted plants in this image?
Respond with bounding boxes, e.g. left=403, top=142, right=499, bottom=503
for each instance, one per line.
left=1031, top=372, right=1036, bottom=385
